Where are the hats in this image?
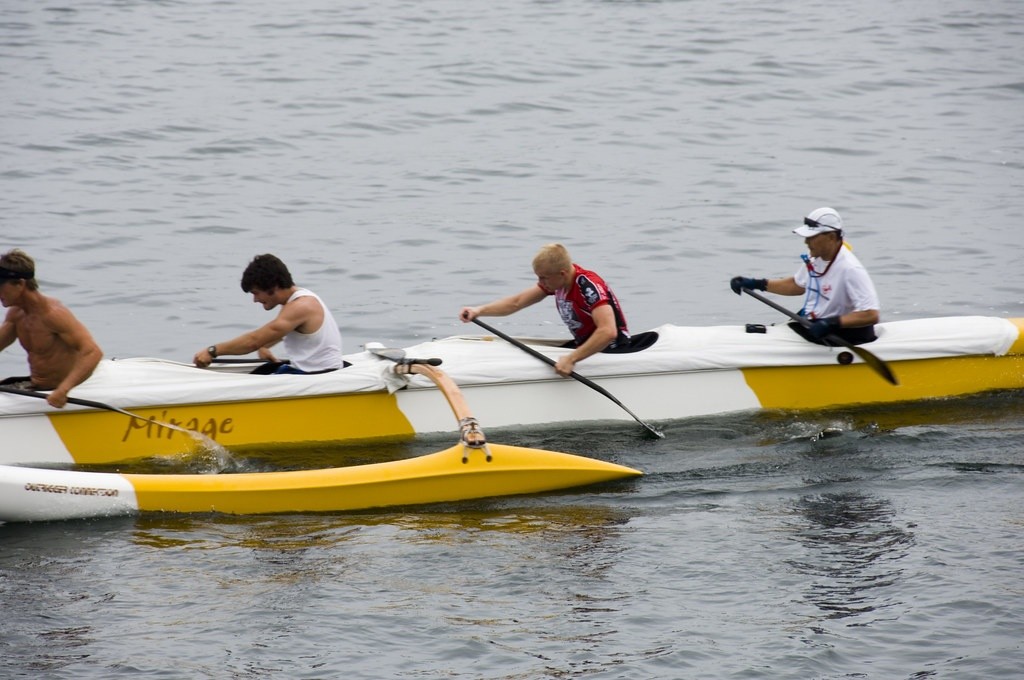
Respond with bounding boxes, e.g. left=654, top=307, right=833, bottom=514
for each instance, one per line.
left=792, top=207, right=841, bottom=238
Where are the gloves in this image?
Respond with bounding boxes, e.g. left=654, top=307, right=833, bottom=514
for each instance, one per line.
left=809, top=316, right=840, bottom=339
left=731, top=276, right=769, bottom=296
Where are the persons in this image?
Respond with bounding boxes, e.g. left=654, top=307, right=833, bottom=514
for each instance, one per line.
left=0, top=252, right=102, bottom=408
left=731, top=207, right=880, bottom=347
left=193, top=254, right=344, bottom=375
left=459, top=244, right=627, bottom=378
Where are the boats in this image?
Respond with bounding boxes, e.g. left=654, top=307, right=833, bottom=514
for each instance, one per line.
left=0, top=311, right=1024, bottom=525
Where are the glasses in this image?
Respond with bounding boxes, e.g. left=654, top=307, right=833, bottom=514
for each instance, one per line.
left=804, top=217, right=839, bottom=230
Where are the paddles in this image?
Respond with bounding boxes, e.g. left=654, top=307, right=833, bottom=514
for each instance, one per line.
left=462, top=311, right=663, bottom=438
left=0, top=386, right=188, bottom=433
left=210, top=358, right=291, bottom=365
left=736, top=280, right=898, bottom=386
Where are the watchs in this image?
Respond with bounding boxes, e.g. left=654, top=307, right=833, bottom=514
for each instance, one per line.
left=208, top=346, right=217, bottom=357
